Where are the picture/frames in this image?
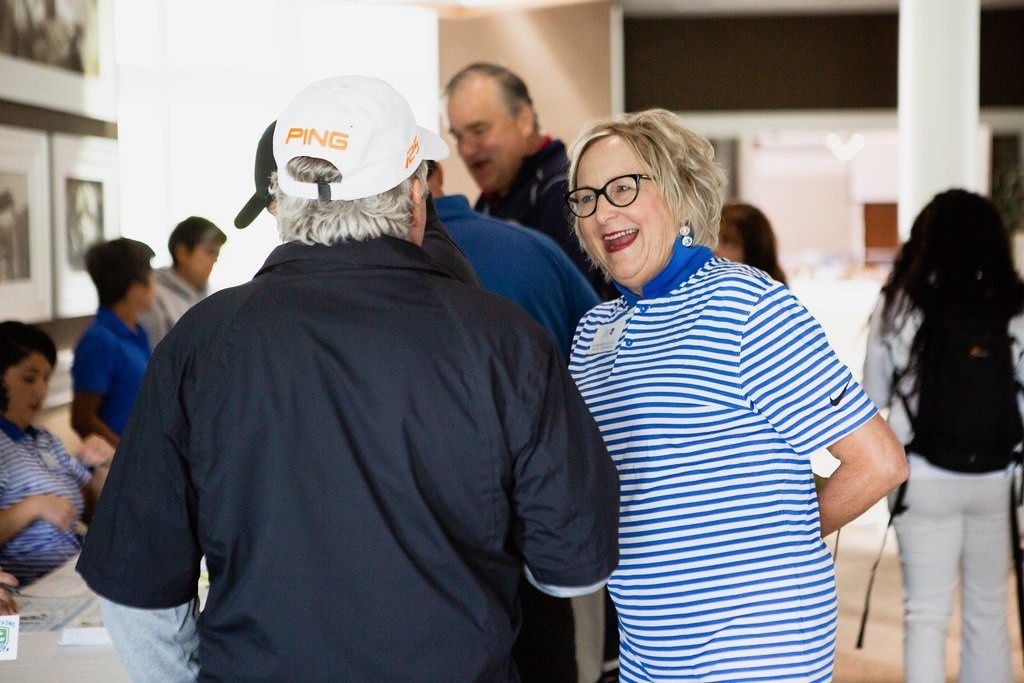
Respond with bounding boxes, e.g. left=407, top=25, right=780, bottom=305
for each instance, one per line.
left=0, top=124, right=54, bottom=324
left=0, top=0, right=119, bottom=124
left=51, top=132, right=117, bottom=321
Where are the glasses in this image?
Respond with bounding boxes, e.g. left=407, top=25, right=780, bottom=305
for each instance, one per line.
left=563, top=173, right=655, bottom=218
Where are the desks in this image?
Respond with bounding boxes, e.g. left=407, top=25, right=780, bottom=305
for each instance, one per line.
left=0, top=554, right=209, bottom=683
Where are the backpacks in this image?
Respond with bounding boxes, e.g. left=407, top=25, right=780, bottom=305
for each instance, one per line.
left=898, top=277, right=1022, bottom=477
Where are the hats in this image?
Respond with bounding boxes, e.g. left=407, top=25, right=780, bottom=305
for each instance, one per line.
left=235, top=120, right=277, bottom=229
left=273, top=74, right=450, bottom=200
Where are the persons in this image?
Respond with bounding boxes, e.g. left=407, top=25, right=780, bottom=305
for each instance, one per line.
left=0, top=321, right=115, bottom=585
left=446, top=64, right=622, bottom=302
left=859, top=189, right=1024, bottom=683
left=567, top=111, right=909, bottom=682
left=69, top=238, right=156, bottom=446
left=141, top=216, right=227, bottom=351
left=73, top=74, right=620, bottom=682
left=235, top=119, right=599, bottom=351
left=715, top=203, right=785, bottom=284
left=0, top=567, right=20, bottom=616
